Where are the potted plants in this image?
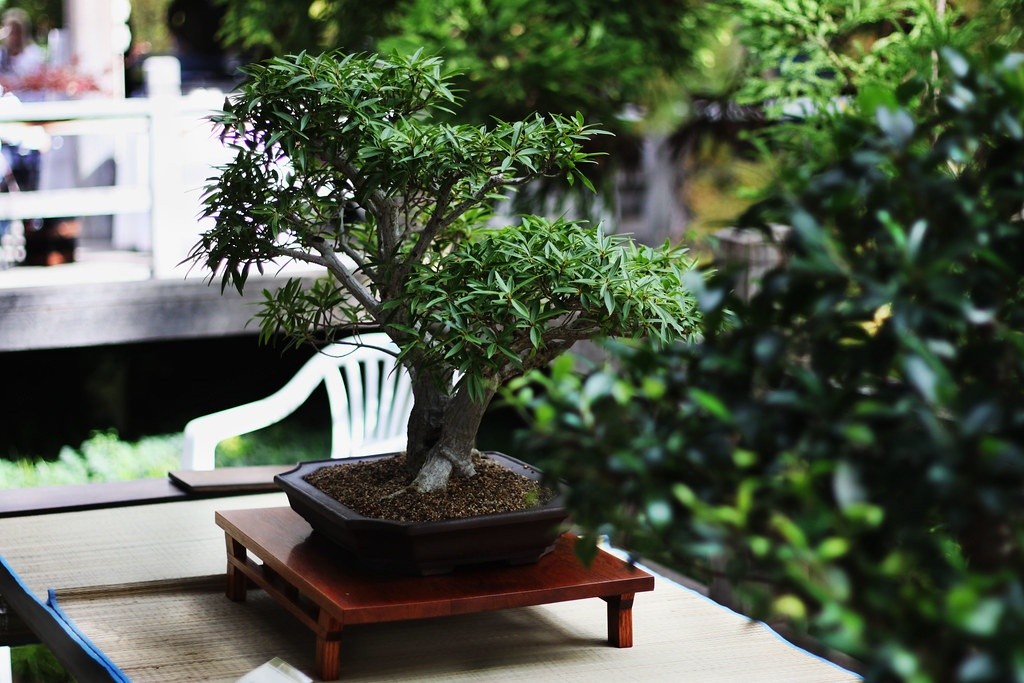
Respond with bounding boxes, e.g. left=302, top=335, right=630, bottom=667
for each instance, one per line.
left=174, top=45, right=707, bottom=570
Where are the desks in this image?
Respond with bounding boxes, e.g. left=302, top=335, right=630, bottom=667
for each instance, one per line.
left=0, top=490, right=866, bottom=683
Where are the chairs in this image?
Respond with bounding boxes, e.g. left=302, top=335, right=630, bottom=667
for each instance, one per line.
left=180, top=331, right=466, bottom=471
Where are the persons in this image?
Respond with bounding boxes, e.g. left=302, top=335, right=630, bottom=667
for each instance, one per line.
left=0, top=0, right=255, bottom=267
